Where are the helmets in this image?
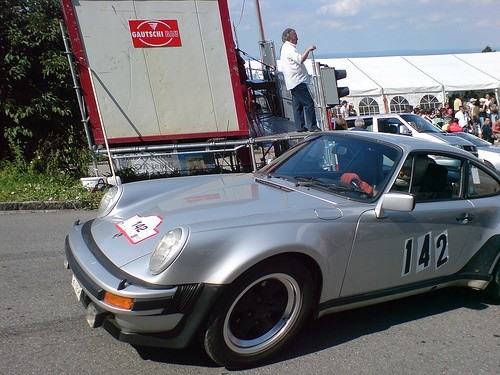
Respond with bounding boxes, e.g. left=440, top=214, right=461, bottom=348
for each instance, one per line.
left=395, top=153, right=429, bottom=186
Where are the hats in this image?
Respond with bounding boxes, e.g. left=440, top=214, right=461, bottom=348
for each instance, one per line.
left=479, top=98, right=488, bottom=102
left=470, top=97, right=477, bottom=101
left=342, top=100, right=348, bottom=104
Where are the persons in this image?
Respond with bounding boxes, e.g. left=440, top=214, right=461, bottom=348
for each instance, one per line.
left=280, top=28, right=322, bottom=131
left=350, top=117, right=368, bottom=131
left=335, top=118, right=347, bottom=131
left=349, top=102, right=357, bottom=116
left=332, top=113, right=338, bottom=118
left=404, top=89, right=499, bottom=144
left=340, top=99, right=349, bottom=117
left=340, top=152, right=428, bottom=198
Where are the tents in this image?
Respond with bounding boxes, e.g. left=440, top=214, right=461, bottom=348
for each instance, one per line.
left=243, top=52, right=500, bottom=116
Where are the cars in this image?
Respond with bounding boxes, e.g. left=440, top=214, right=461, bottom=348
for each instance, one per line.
left=450, top=132, right=500, bottom=171
left=65, top=132, right=500, bottom=366
left=345, top=113, right=478, bottom=158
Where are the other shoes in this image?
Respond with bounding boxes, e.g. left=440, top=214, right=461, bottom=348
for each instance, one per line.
left=311, top=126, right=322, bottom=132
left=298, top=127, right=308, bottom=131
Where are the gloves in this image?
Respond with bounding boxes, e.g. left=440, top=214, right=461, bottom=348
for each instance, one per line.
left=341, top=173, right=373, bottom=194
